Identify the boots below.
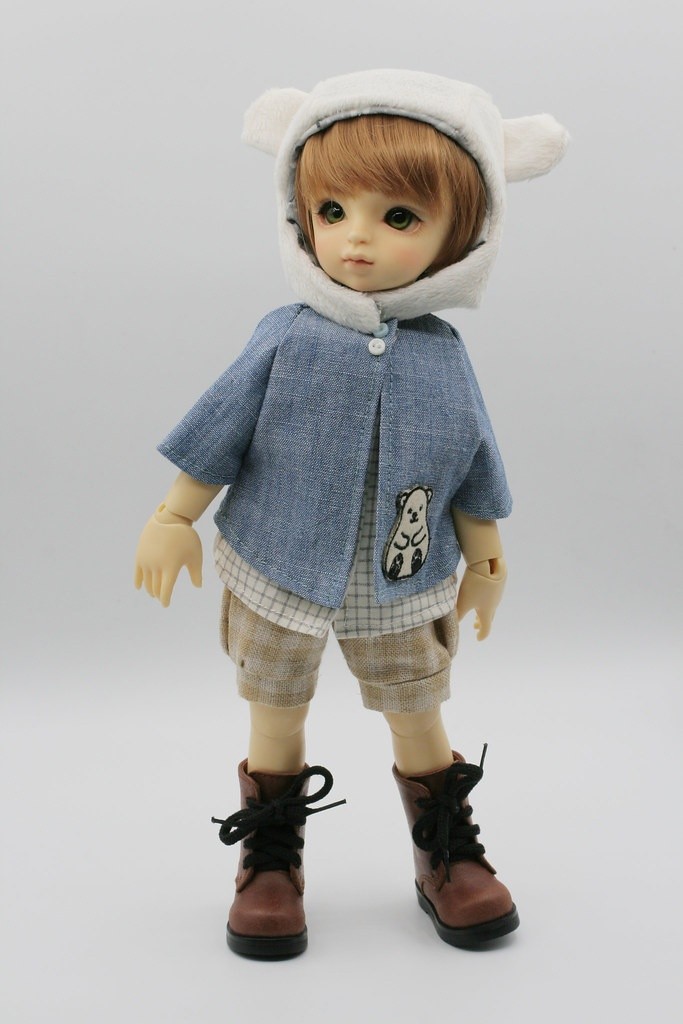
[391,743,520,948]
[210,759,347,960]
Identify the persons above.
[134,67,570,963]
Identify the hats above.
[241,67,568,334]
